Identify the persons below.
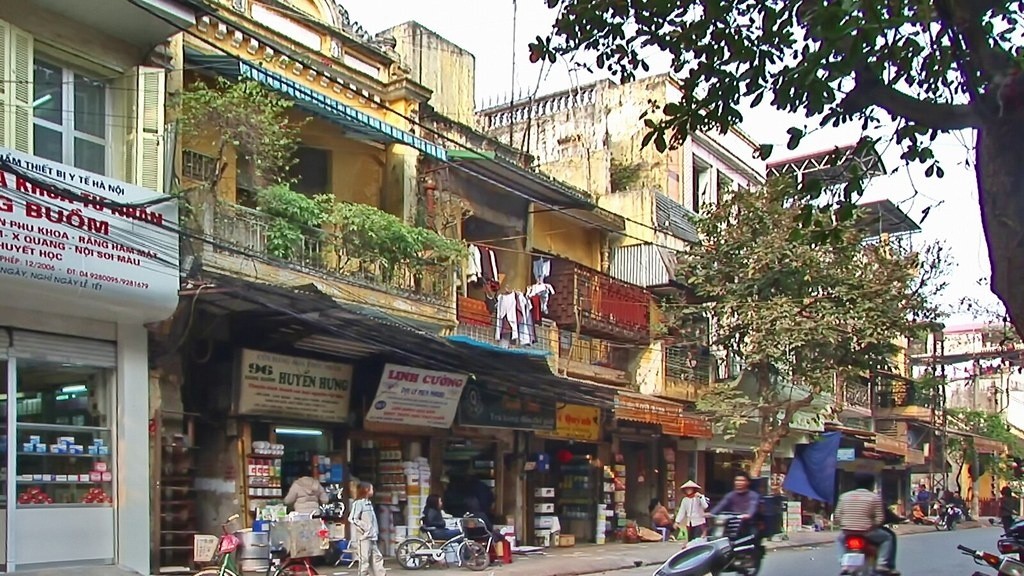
[997,486,1018,543]
[703,472,759,565]
[348,481,387,576]
[283,466,329,516]
[834,472,895,574]
[649,498,680,532]
[917,484,930,516]
[422,495,470,560]
[673,479,710,543]
[465,496,496,566]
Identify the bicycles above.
[935,504,959,530]
[190,514,321,576]
[396,512,492,570]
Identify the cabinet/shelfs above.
[15,421,112,509]
[556,453,595,519]
[247,453,283,512]
[154,409,200,576]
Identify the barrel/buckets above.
[596,503,607,544]
[235,532,269,571]
[378,456,432,559]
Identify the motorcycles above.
[839,535,880,576]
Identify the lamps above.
[274,427,322,436]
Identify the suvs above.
[702,514,766,576]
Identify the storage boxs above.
[535,487,555,497]
[534,503,554,513]
[534,516,553,528]
[559,534,575,547]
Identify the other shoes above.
[876,564,890,571]
[744,559,754,566]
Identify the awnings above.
[187,53,448,161]
[917,420,1007,454]
[449,151,599,209]
[780,433,841,503]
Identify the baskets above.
[193,533,221,564]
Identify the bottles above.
[558,473,587,519]
[360,439,407,504]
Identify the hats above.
[680,480,702,489]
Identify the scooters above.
[957,544,1024,576]
[997,519,1024,563]
[652,534,754,576]
